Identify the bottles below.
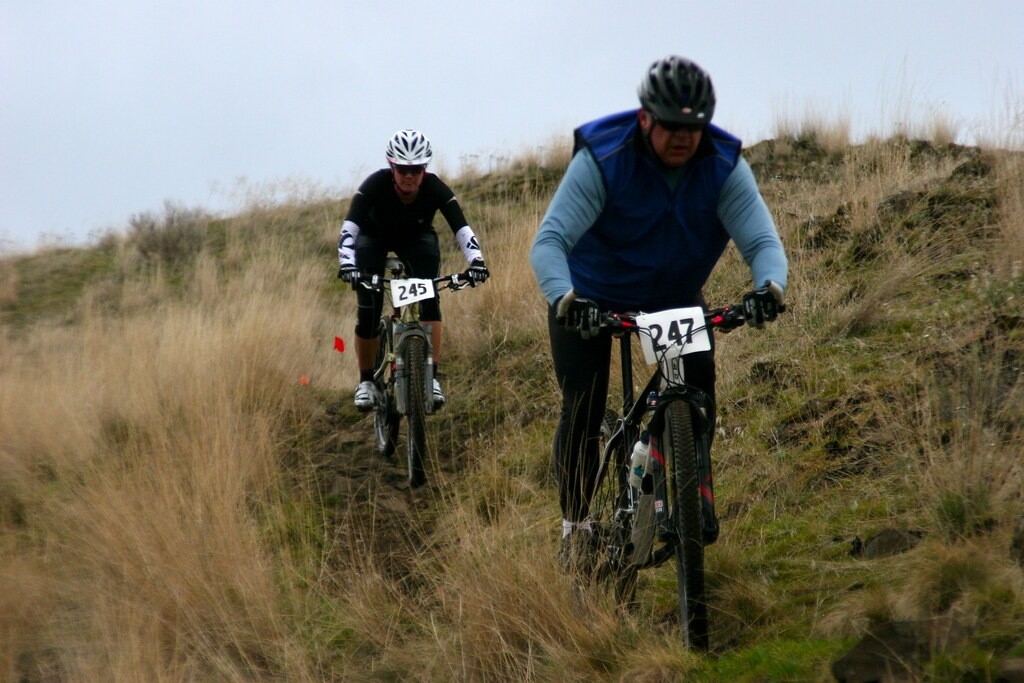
[629,429,650,488]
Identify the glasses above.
[644,106,710,134]
[390,163,425,176]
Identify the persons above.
[529,54,790,578]
[338,130,490,411]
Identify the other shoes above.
[559,526,594,572]
[658,516,682,544]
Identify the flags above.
[334,336,344,352]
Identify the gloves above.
[465,264,489,288]
[339,264,362,291]
[563,298,600,339]
[743,286,780,331]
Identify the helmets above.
[386,128,432,165]
[639,55,715,124]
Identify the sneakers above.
[353,380,377,408]
[423,378,446,405]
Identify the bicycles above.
[348,260,479,490]
[567,300,787,655]
